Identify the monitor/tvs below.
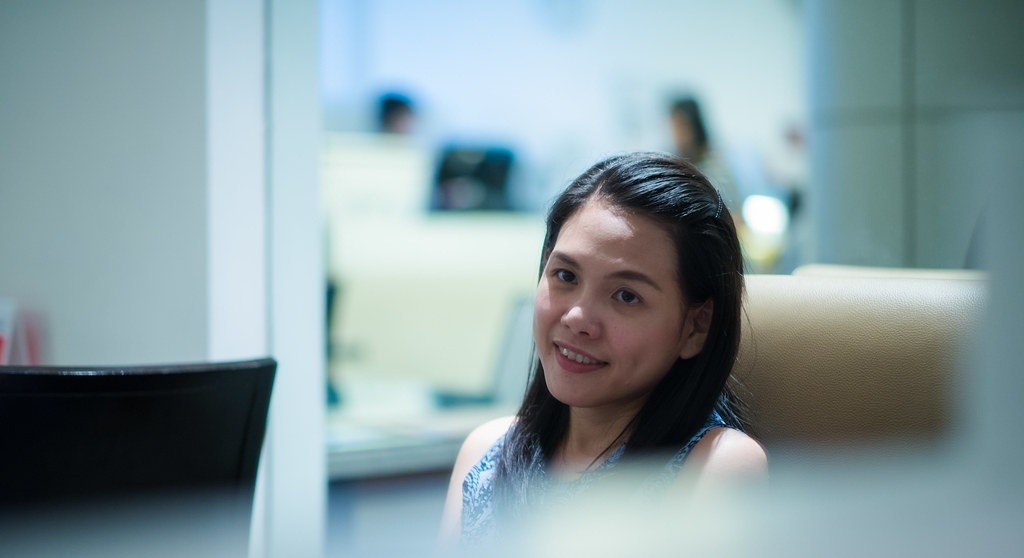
[0,357,278,558]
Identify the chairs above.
[697,270,998,557]
[1,358,277,558]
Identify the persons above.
[374,95,416,134]
[440,150,769,558]
[664,89,742,228]
